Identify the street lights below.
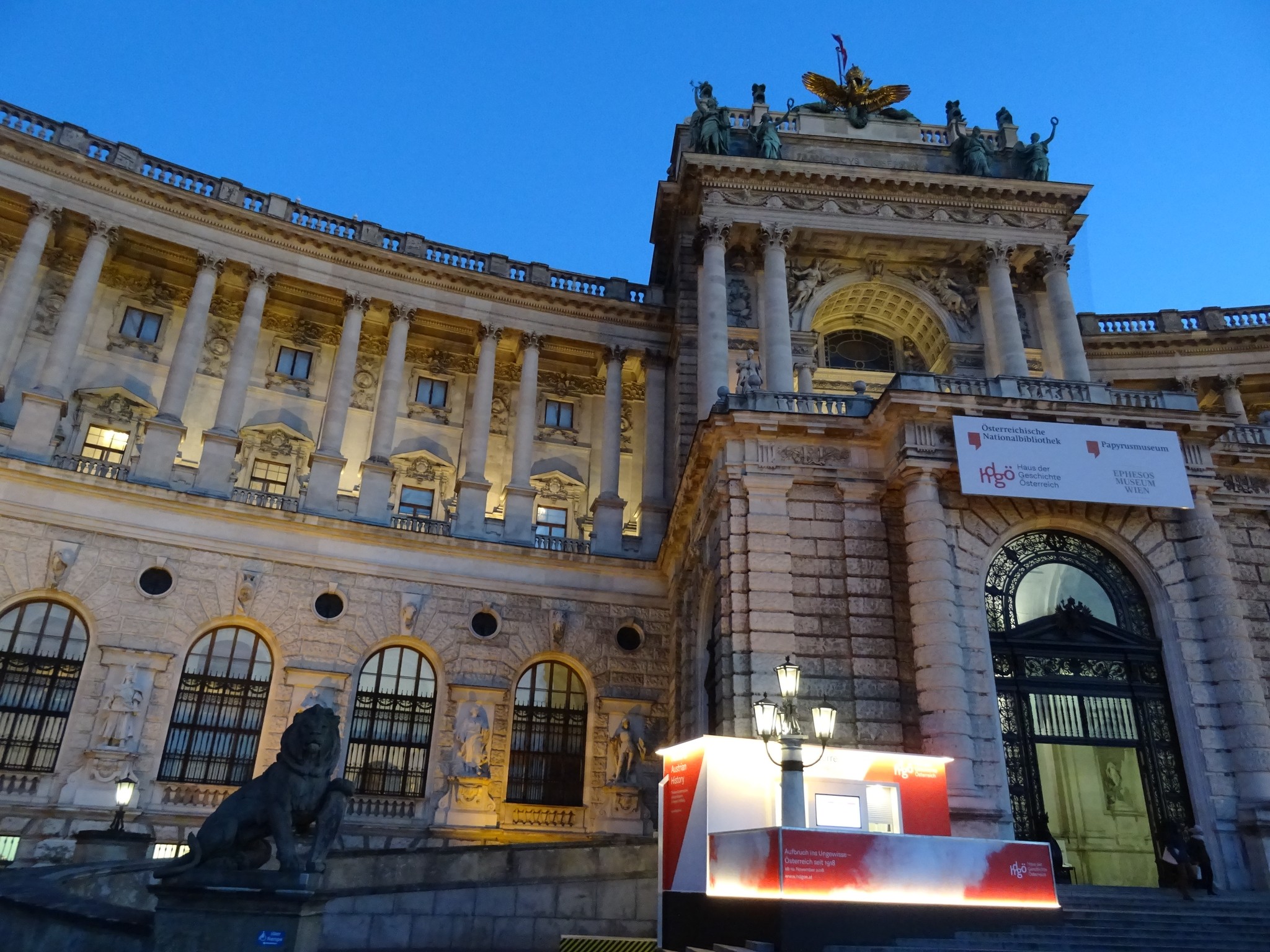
[752,656,838,830]
[105,772,137,830]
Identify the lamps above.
[106,771,137,833]
[752,654,837,831]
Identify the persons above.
[1165,824,1195,900]
[97,670,143,748]
[757,104,791,160]
[735,349,762,393]
[913,267,969,313]
[736,358,750,385]
[1026,124,1056,181]
[694,87,727,154]
[786,260,841,314]
[1189,825,1217,896]
[611,717,637,785]
[456,708,486,775]
[955,117,994,177]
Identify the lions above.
[152,703,357,879]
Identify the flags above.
[831,33,847,72]
